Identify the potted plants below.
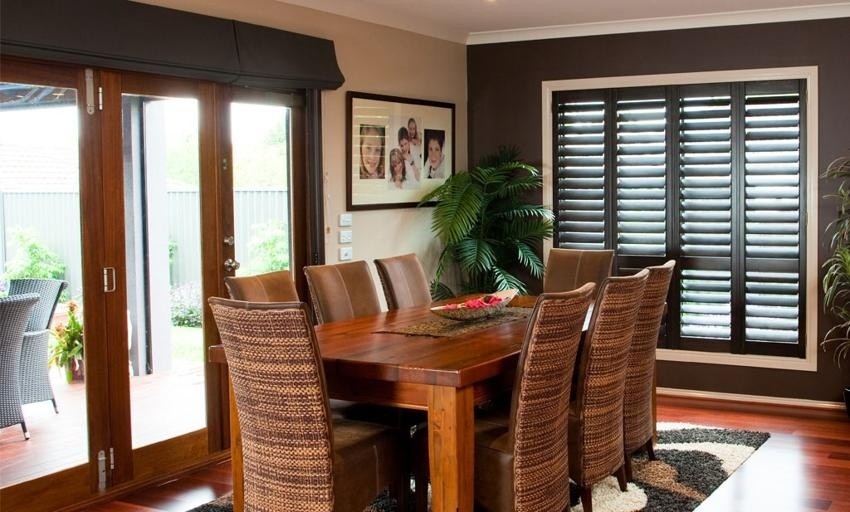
[818,155,850,418]
[49,300,87,385]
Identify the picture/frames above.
[344,90,460,211]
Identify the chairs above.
[0,291,41,440]
[221,252,435,337]
[9,279,68,413]
[541,246,623,297]
[205,296,401,512]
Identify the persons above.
[408,118,422,144]
[360,124,385,179]
[398,127,421,181]
[390,148,405,187]
[424,129,445,178]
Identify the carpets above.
[185,418,771,512]
